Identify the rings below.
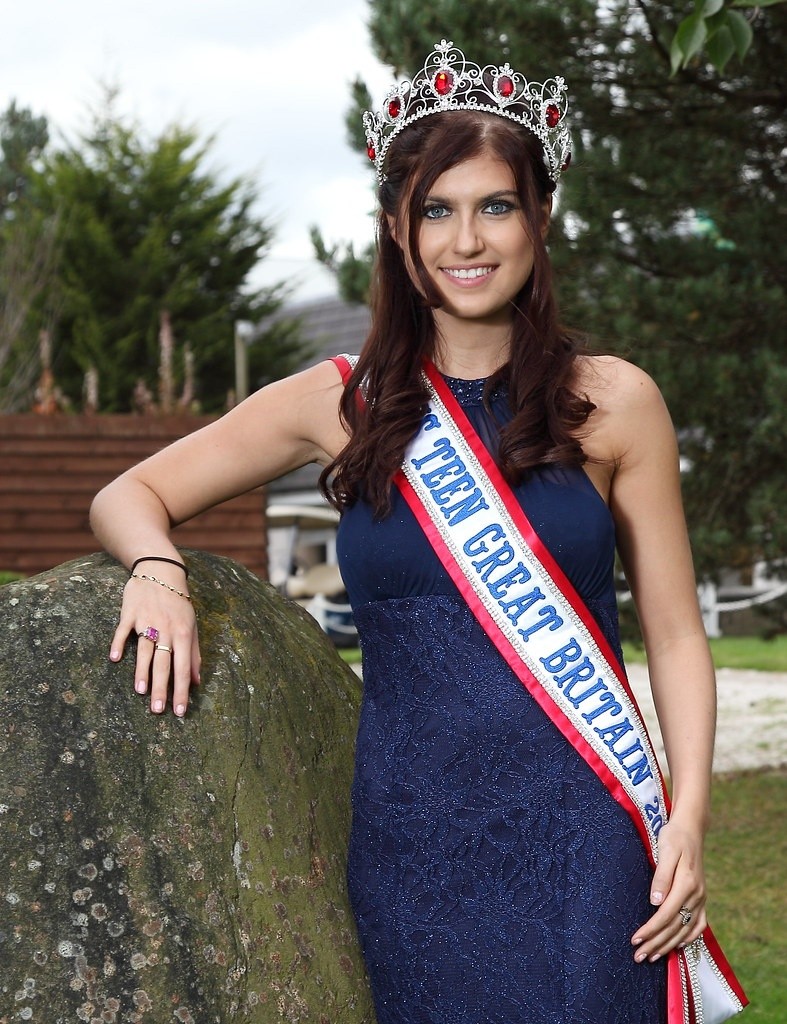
[139,626,158,642]
[679,907,692,925]
[154,645,172,654]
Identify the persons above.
[88,38,717,1024]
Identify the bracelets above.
[130,573,192,601]
[130,556,189,581]
